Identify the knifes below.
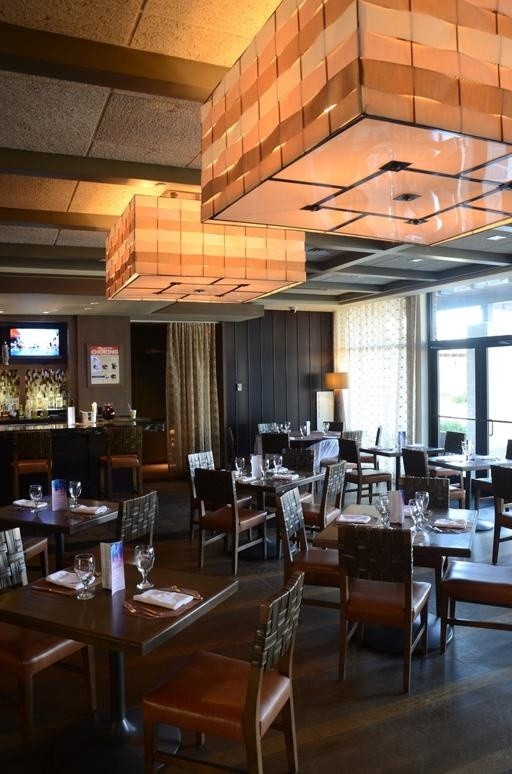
[31,582,74,595]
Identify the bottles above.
[103,405,115,419]
[1,368,68,418]
[91,402,98,416]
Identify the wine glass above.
[30,485,44,514]
[272,455,282,470]
[274,420,330,434]
[372,489,430,532]
[69,481,81,509]
[234,457,245,478]
[75,554,96,602]
[460,440,475,462]
[260,458,269,479]
[134,545,155,590]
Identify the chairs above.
[275,485,341,608]
[335,522,433,695]
[440,559,512,656]
[2,418,512,576]
[2,526,97,741]
[141,568,305,772]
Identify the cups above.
[91,412,96,422]
[98,407,102,414]
[80,412,88,421]
[129,410,137,419]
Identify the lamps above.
[324,370,351,394]
[97,185,310,310]
[188,0,512,255]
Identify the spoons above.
[122,600,162,618]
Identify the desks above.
[311,503,480,659]
[2,554,240,773]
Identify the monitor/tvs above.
[6,323,70,367]
